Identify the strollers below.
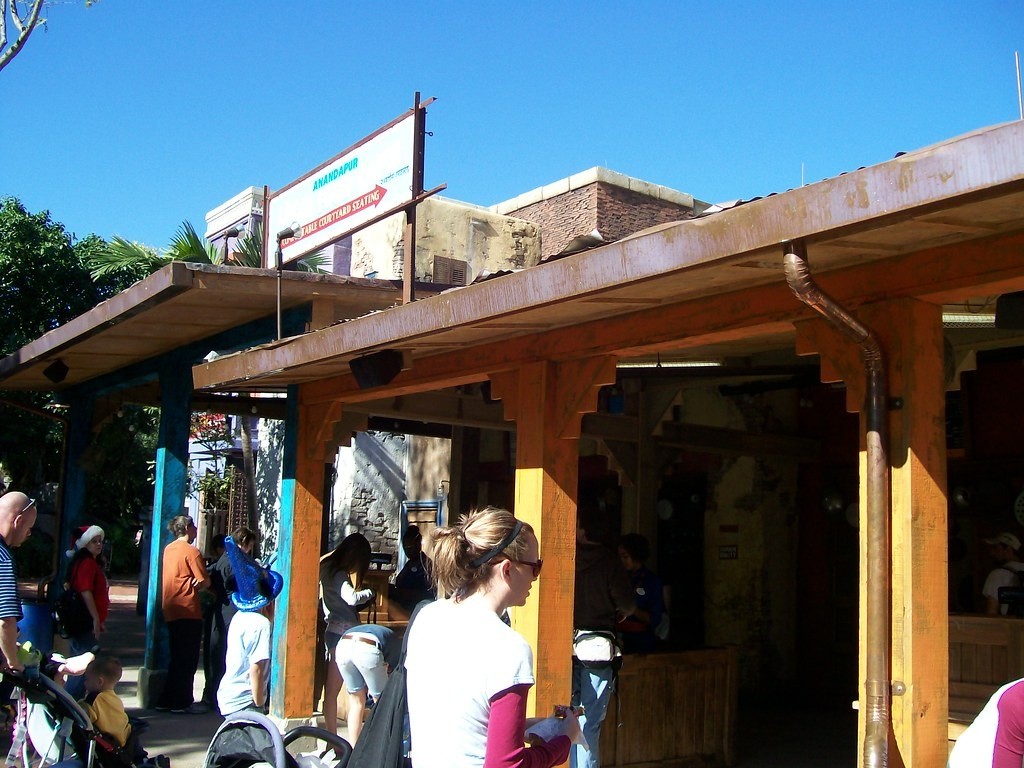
[2,641,152,768]
[204,712,353,768]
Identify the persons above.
[155,516,211,714]
[217,536,284,721]
[319,533,376,751]
[405,505,589,768]
[982,532,1024,617]
[0,491,132,768]
[570,512,672,768]
[945,678,1024,768]
[335,623,401,749]
[388,525,438,618]
[201,527,257,712]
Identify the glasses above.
[490,558,543,577]
[13,498,38,523]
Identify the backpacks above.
[52,556,97,640]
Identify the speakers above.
[347,347,406,391]
[42,358,71,383]
[994,289,1024,329]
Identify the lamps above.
[43,362,68,383]
[349,347,415,388]
[275,222,303,339]
[220,223,247,263]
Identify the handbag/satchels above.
[346,598,434,768]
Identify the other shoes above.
[154,704,188,713]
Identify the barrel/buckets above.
[15,597,54,657]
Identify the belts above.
[342,634,381,650]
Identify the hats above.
[65,524,105,559]
[224,536,284,612]
